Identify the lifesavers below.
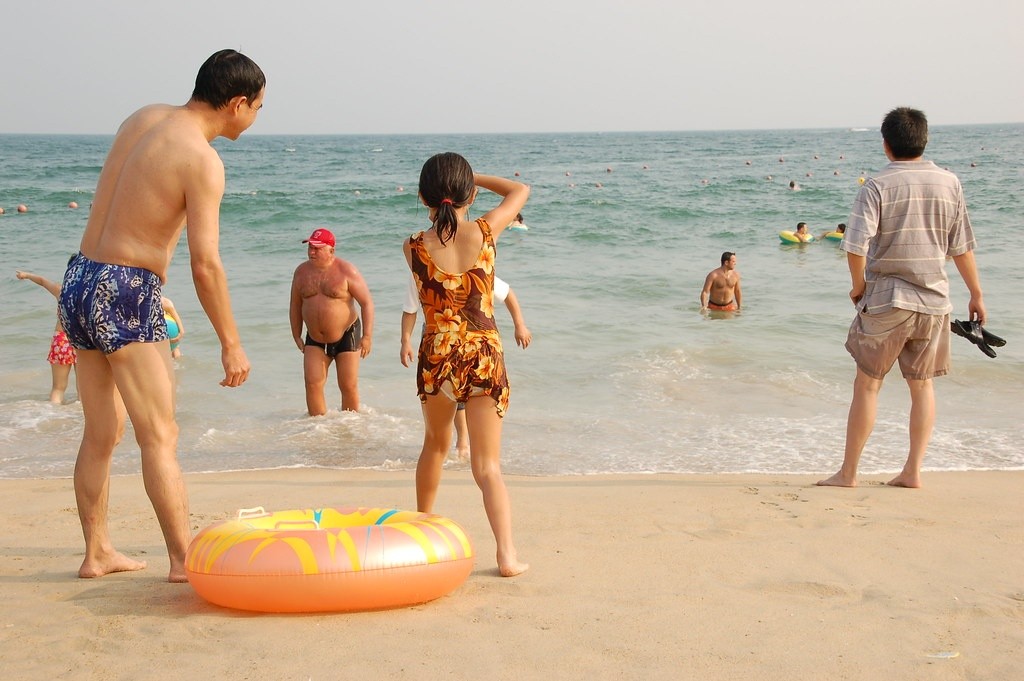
[506,222,528,231]
[777,229,813,247]
[182,507,472,613]
[163,315,181,350]
[824,232,844,242]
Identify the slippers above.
[951,319,1006,358]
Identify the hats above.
[301,229,336,249]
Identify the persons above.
[290,229,373,417]
[700,251,742,309]
[160,298,184,359]
[59,49,266,583]
[403,152,530,574]
[16,270,80,403]
[817,107,986,488]
[400,268,532,449]
[0,202,79,214]
[354,155,978,243]
